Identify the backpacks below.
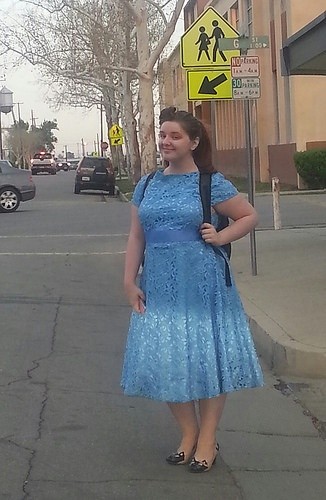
[144,172,231,260]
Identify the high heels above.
[166,443,197,465]
[189,441,220,473]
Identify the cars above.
[0,163,35,213]
[57,159,78,172]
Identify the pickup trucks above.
[31,153,58,174]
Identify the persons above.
[123,107,265,473]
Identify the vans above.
[74,156,116,194]
